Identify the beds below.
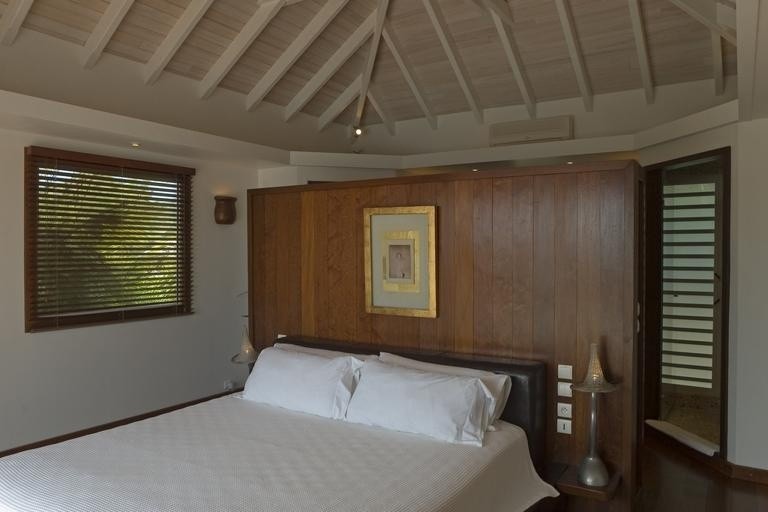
[0,334,546,512]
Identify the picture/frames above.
[362,205,437,319]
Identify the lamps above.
[214,195,238,224]
[230,326,260,374]
[570,341,620,487]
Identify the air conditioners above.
[489,115,574,146]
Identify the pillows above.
[379,351,512,432]
[243,347,364,421]
[345,358,496,448]
[273,343,379,394]
